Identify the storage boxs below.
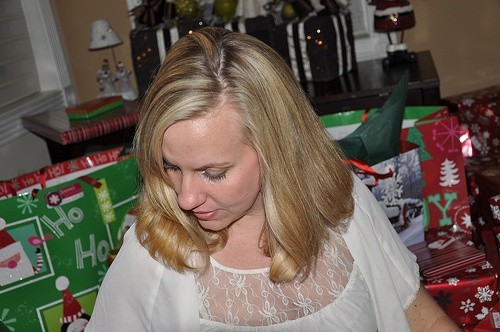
[65,95,124,120]
[406,85,500,332]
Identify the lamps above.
[88,19,138,101]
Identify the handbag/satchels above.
[0,146,142,332]
[317,105,474,248]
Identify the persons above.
[83,27,460,332]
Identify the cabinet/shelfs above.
[299,49,442,117]
[21,100,142,165]
